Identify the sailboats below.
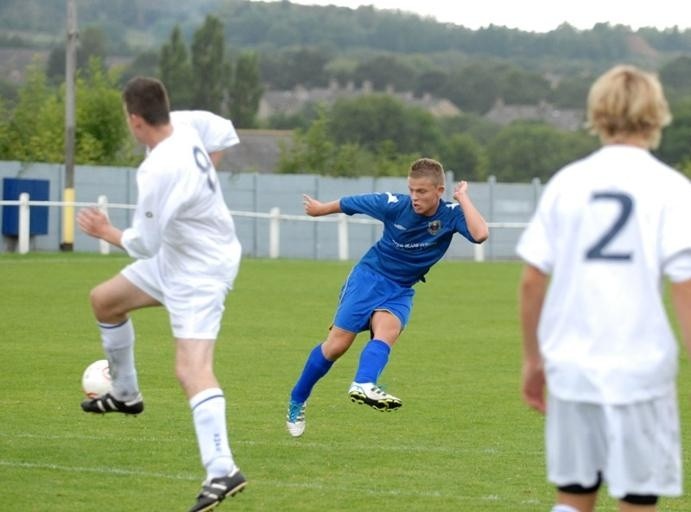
[81,359,113,401]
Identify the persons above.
[77,76,248,512]
[515,65,690,511]
[287,158,489,437]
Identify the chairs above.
[189,469,246,512]
[287,400,306,437]
[80,392,144,414]
[348,382,403,412]
